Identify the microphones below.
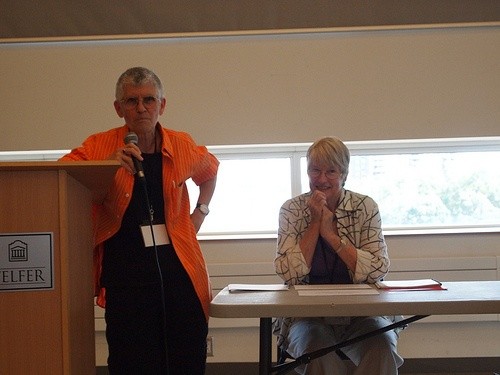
[123,132,146,181]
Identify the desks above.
[210,280,500,375]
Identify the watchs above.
[336,238,347,253]
[196,203,209,215]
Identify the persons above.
[273,137,403,375]
[56,67,219,374]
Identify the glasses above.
[122,95,162,110]
[307,167,343,180]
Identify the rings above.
[121,147,126,154]
[121,154,126,161]
